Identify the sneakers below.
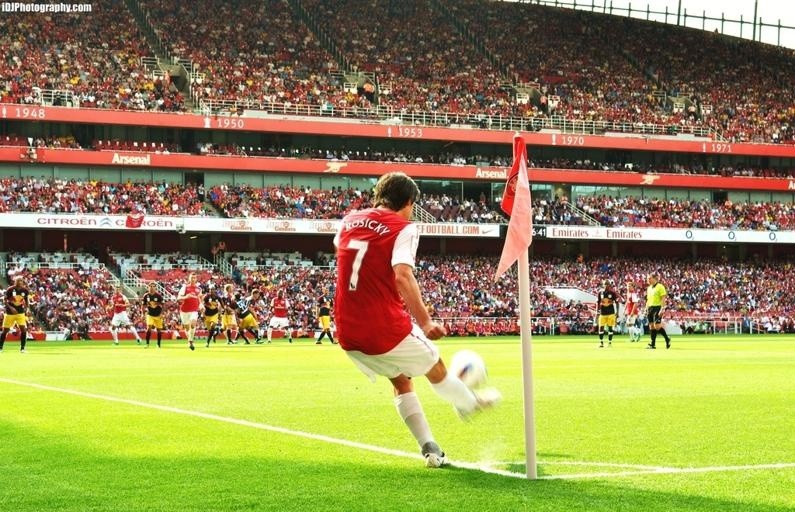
[0,336,341,354]
[599,336,642,350]
[425,448,450,469]
[665,335,672,350]
[644,343,657,350]
[454,384,501,425]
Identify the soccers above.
[451,351,485,386]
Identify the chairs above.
[0,0,795,337]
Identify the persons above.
[1,1,795,352]
[333,171,499,468]
